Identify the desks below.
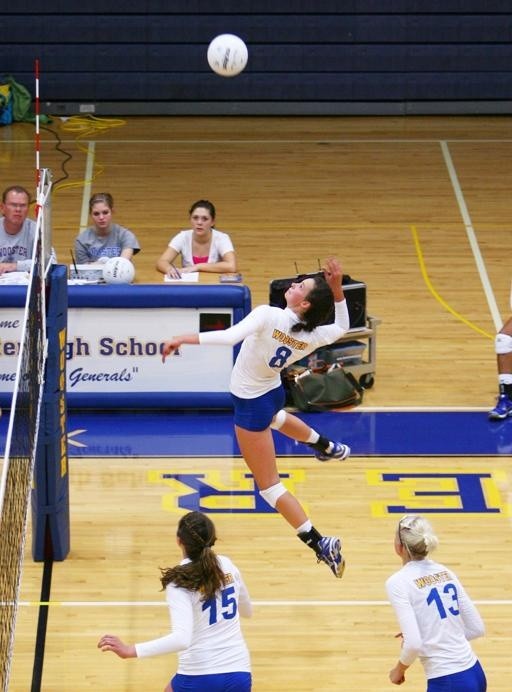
[1,270,251,409]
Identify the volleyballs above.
[102,257,134,285]
[208,33,248,77]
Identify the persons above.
[156,199,237,280]
[96,513,254,692]
[384,515,487,692]
[74,192,141,266]
[161,257,351,578]
[488,315,512,422]
[0,186,57,277]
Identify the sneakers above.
[316,442,351,462]
[317,536,346,579]
[489,394,512,420]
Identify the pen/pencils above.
[71,249,77,274]
[172,264,181,279]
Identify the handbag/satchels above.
[289,359,364,412]
